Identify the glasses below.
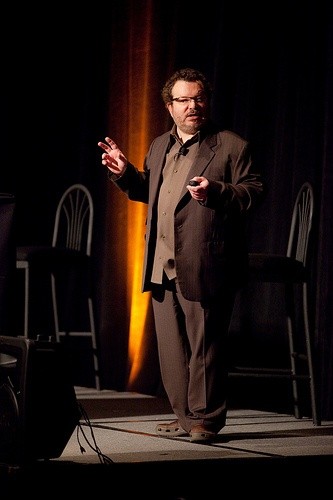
[172,96,208,103]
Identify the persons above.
[99,69,262,441]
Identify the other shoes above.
[190,426,216,442]
[155,420,188,436]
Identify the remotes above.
[189,181,198,186]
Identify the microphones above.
[180,147,186,154]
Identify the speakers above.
[0,336,82,460]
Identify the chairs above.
[223,182,321,427]
[15,184,104,391]
